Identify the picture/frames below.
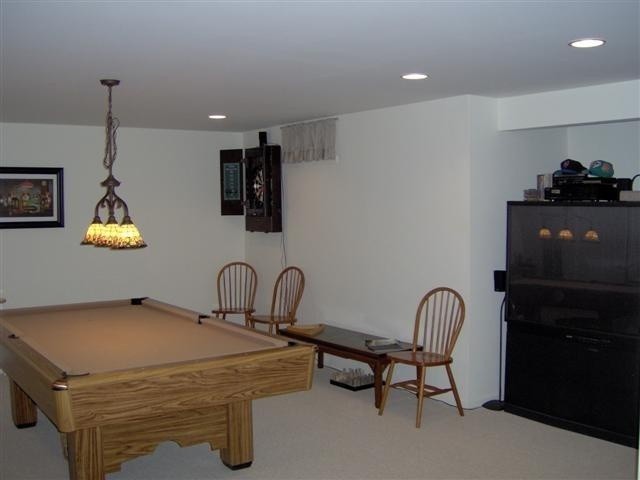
[0,167,65,228]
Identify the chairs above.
[249,263,306,336]
[377,288,465,426]
[211,264,259,324]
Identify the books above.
[365,337,401,346]
[369,343,404,352]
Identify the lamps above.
[80,78,148,250]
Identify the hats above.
[560,159,614,178]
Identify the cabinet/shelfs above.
[504,321,640,450]
[239,143,283,233]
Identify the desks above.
[0,296,318,480]
[281,322,424,407]
